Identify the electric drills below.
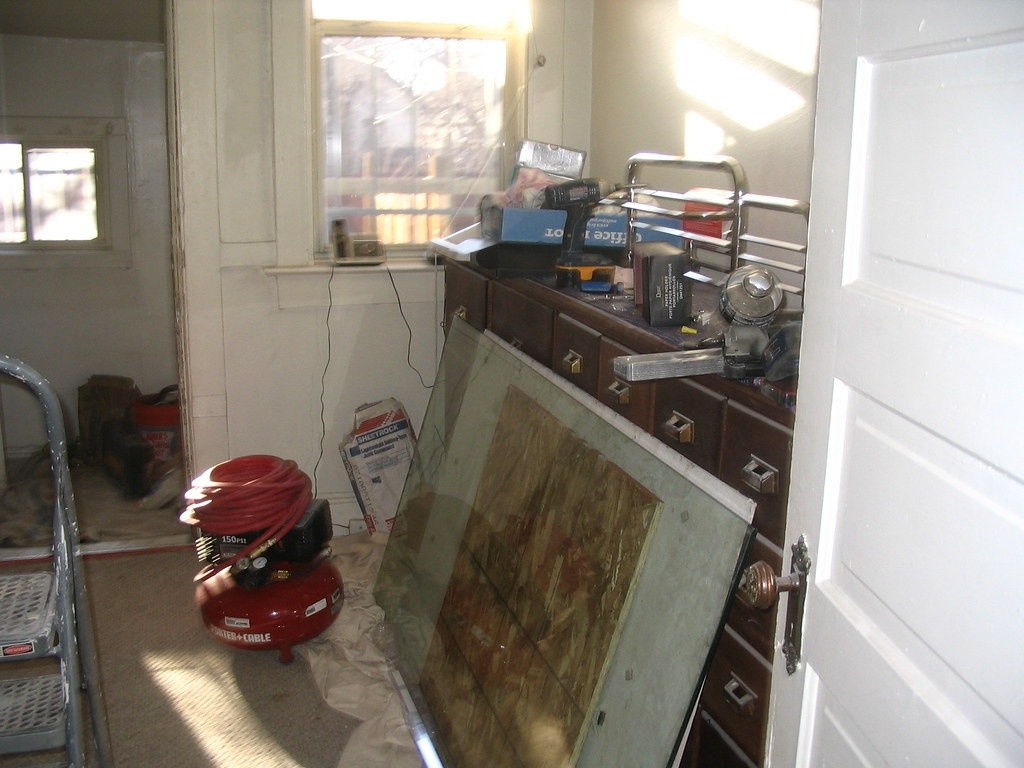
[545,177,648,284]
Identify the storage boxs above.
[556,266,634,288]
[499,209,684,250]
[634,243,693,327]
[339,397,417,535]
[512,139,586,192]
[683,189,741,242]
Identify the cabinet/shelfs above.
[441,256,801,768]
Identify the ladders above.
[0,355,116,768]
